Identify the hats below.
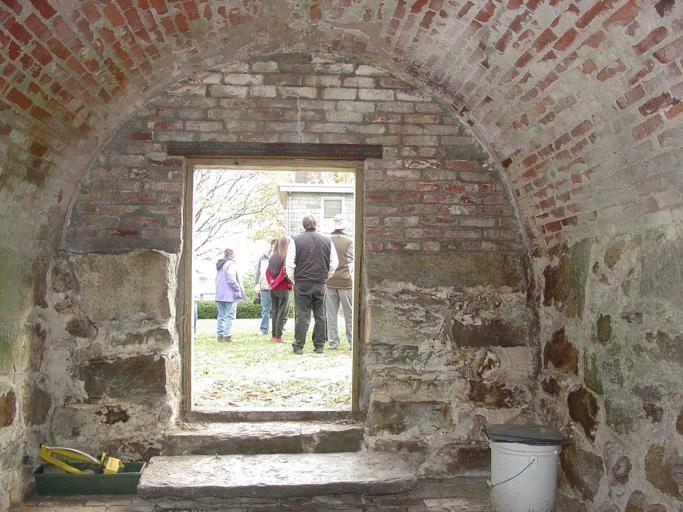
[332,214,346,230]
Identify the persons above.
[265,235,292,343]
[284,214,340,355]
[254,239,277,336]
[213,248,246,342]
[325,213,352,350]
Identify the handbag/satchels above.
[253,291,261,305]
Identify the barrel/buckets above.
[488,441,561,511]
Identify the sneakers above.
[273,338,287,343]
[313,346,322,352]
[255,330,268,336]
[293,347,302,354]
